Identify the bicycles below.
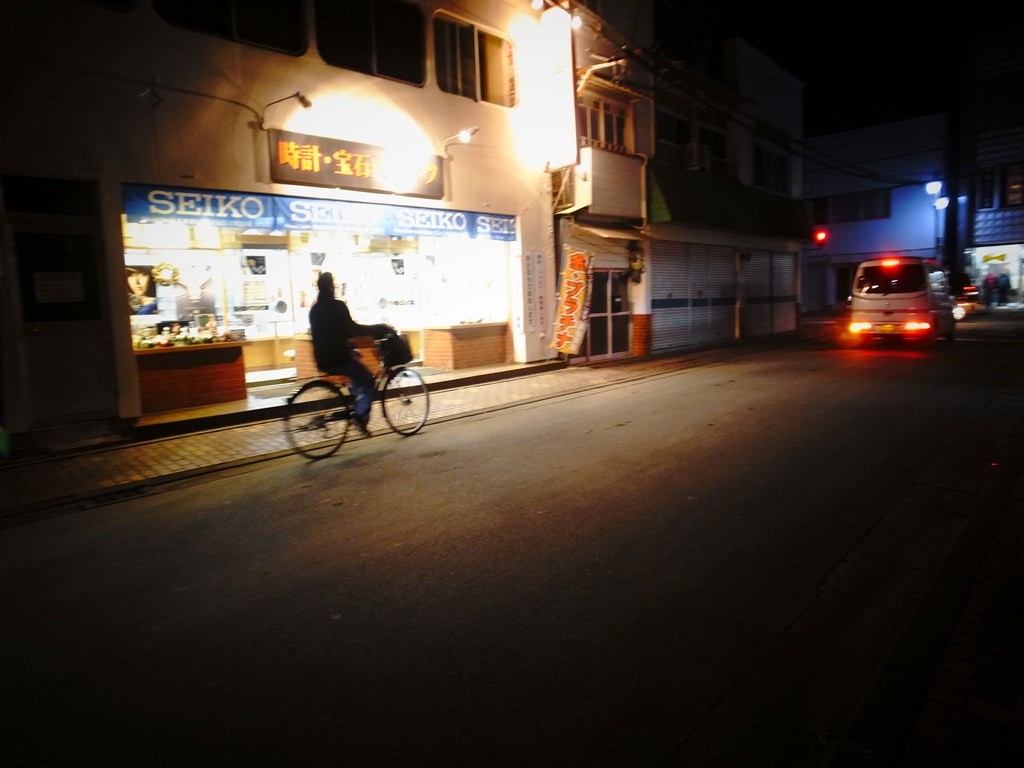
[283,328,430,460]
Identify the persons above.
[125,265,157,315]
[983,273,1010,306]
[309,272,396,439]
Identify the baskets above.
[380,334,413,367]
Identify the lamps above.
[264,91,312,108]
[441,125,479,161]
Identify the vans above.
[849,256,955,345]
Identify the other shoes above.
[347,421,372,440]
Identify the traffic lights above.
[816,227,826,246]
